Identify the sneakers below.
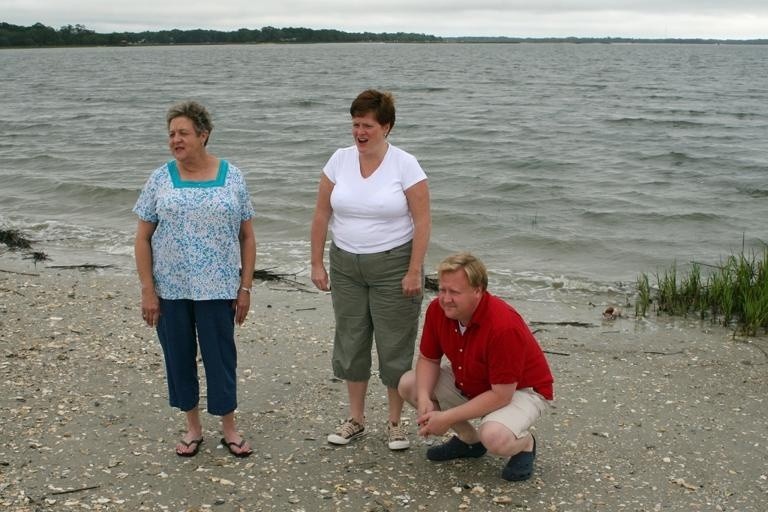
[386,419,410,451]
[500,433,537,482]
[326,416,367,445]
[426,435,488,461]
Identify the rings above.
[143,314,146,318]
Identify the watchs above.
[240,286,253,294]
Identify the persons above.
[131,101,256,458]
[398,254,554,480]
[310,88,431,451]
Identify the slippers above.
[176,437,204,457]
[220,437,253,458]
